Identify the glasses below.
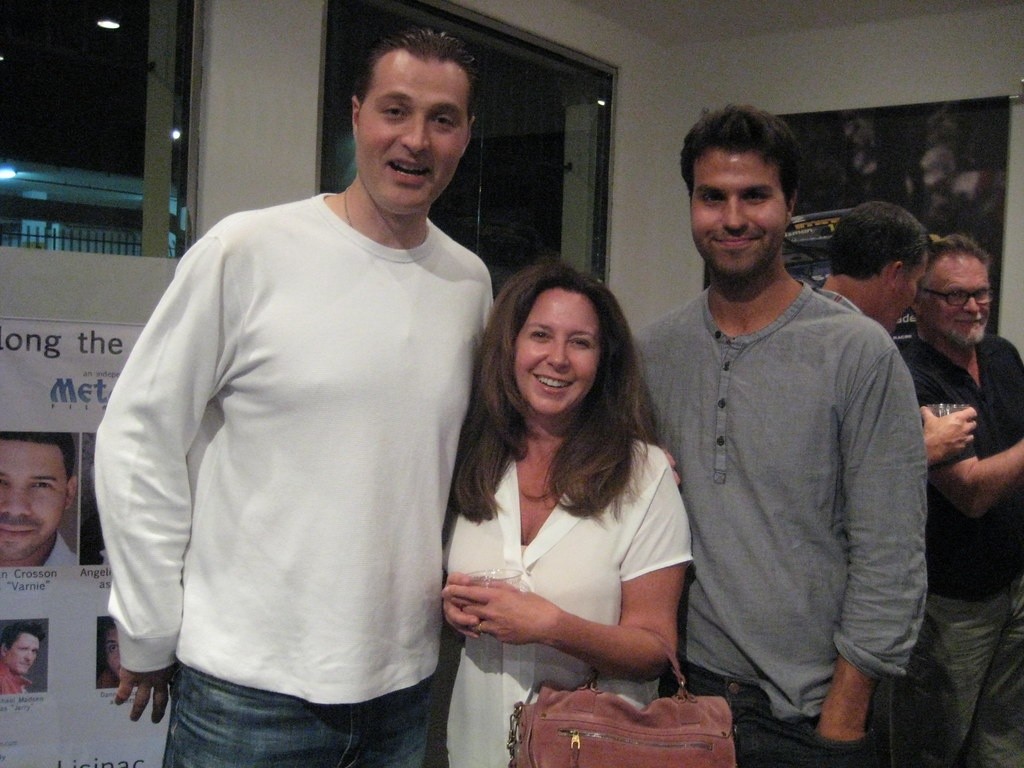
[923,288,994,307]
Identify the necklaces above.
[344,187,353,228]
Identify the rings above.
[476,622,487,634]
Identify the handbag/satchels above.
[505,623,737,768]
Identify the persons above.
[97,624,122,688]
[890,233,1024,768]
[633,102,927,768]
[0,620,46,694]
[0,431,77,566]
[443,264,694,767]
[94,30,493,768]
[803,201,977,467]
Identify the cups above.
[927,404,969,456]
[461,569,523,639]
